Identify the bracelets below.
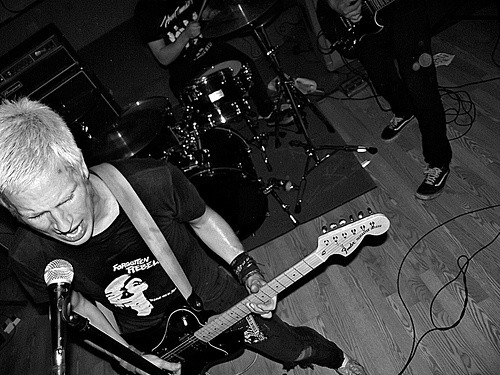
[229,251,261,283]
[121,344,145,374]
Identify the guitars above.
[316,0,394,60]
[150,207,391,375]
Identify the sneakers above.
[416,164,450,200]
[381,113,417,141]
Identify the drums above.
[198,61,254,90]
[179,67,251,128]
[122,97,173,127]
[162,127,269,242]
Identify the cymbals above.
[93,108,162,162]
[201,0,275,39]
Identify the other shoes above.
[266,110,296,127]
[334,352,368,375]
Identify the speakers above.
[32,64,122,168]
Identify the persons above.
[139,0,295,126]
[0,96,368,375]
[314,0,453,199]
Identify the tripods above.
[232,0,378,214]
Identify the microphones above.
[44,258,74,375]
